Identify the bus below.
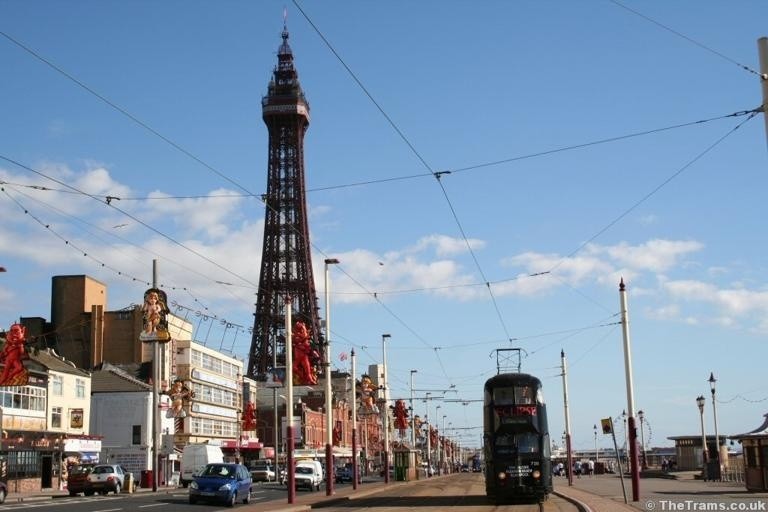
[484,373,553,503]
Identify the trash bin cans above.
[141,469,153,488]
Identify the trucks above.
[473,455,481,472]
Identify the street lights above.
[707,372,721,454]
[381,332,391,483]
[324,257,340,494]
[695,395,707,457]
[406,369,458,481]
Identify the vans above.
[294,459,323,491]
[179,446,227,487]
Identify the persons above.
[332,374,444,452]
[587,457,595,477]
[575,458,582,479]
[143,292,161,334]
[558,461,563,476]
[0,324,26,384]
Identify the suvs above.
[189,464,254,506]
[336,463,363,485]
[461,464,469,472]
[68,463,96,497]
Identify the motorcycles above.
[278,468,287,486]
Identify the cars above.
[248,459,274,482]
[86,464,136,494]
[0,482,6,506]
